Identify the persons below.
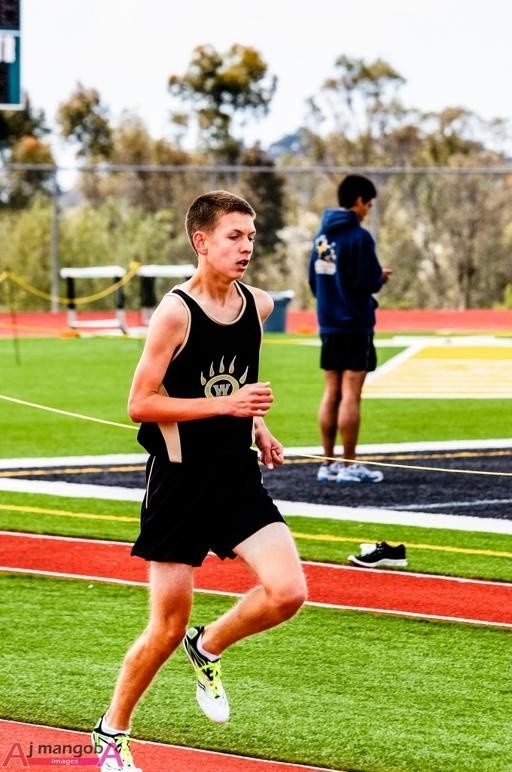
[91,189,309,771]
[307,175,392,485]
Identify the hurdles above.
[136,264,196,326]
[60,265,128,335]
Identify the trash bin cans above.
[263,297,291,332]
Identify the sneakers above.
[347,541,408,568]
[91,713,137,772]
[316,461,384,484]
[183,626,230,723]
